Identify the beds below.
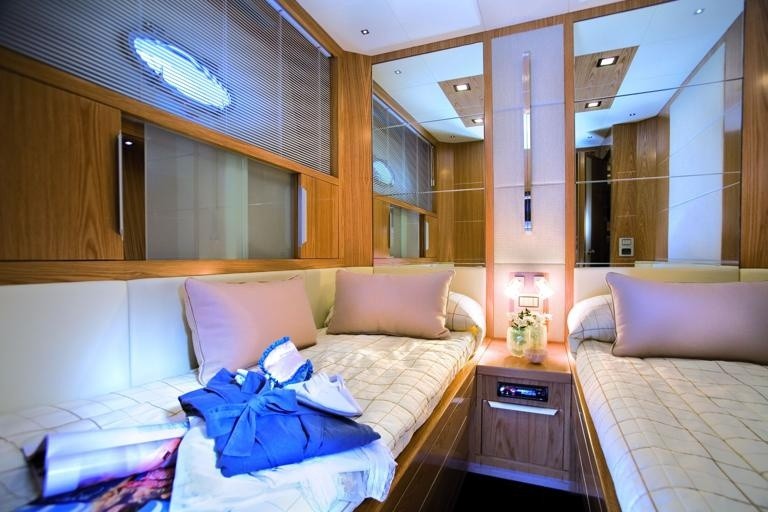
[566,337,768,511]
[2,330,483,512]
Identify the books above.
[18,420,190,496]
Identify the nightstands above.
[470,337,573,481]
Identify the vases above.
[507,327,548,363]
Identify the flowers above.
[508,308,553,329]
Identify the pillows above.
[322,268,485,344]
[566,271,767,364]
[181,274,318,381]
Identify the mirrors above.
[370,41,486,267]
[571,1,745,267]
